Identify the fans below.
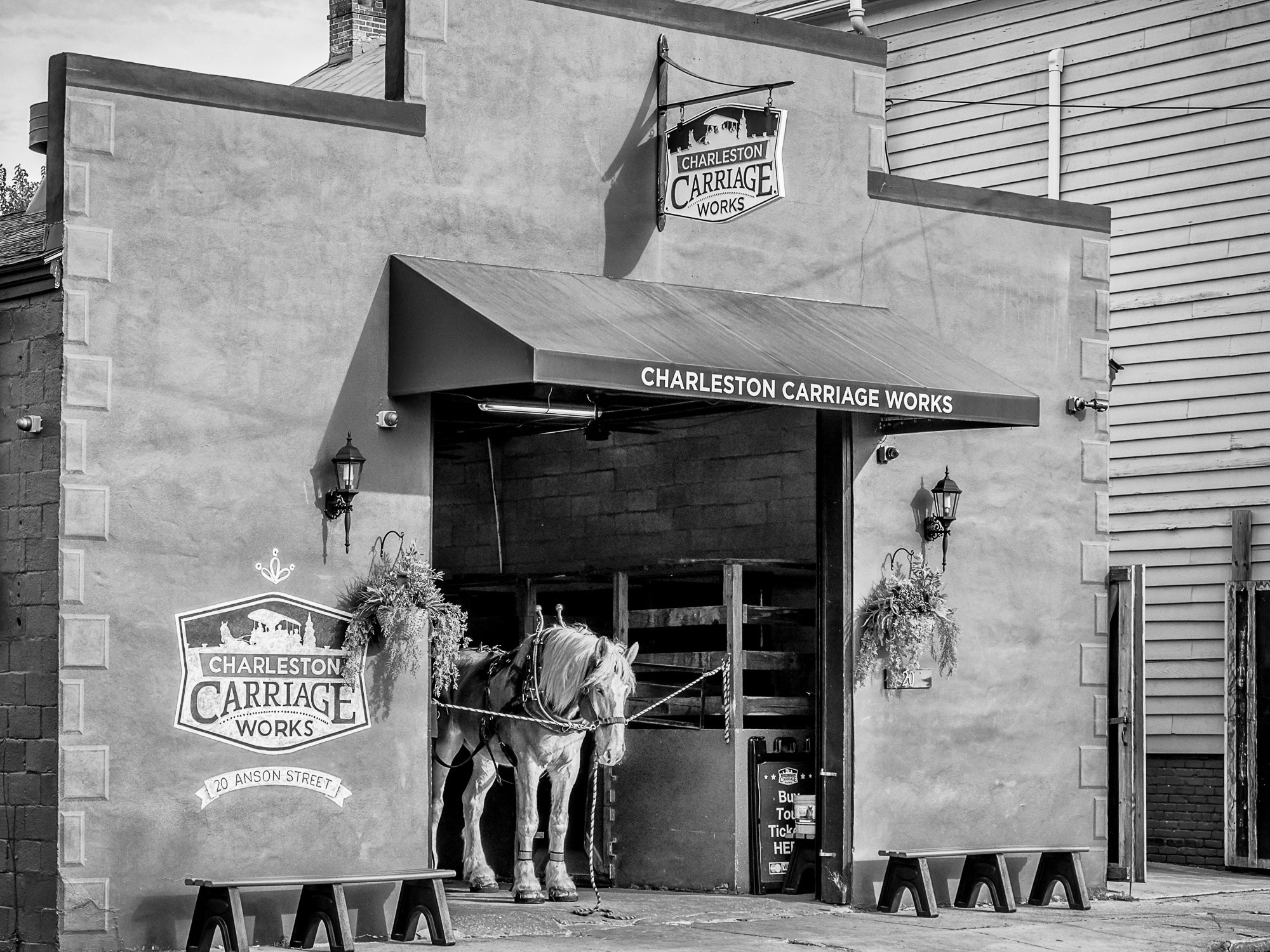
[525,390,661,441]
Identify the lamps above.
[924,465,962,571]
[478,386,603,418]
[325,430,366,554]
[1069,396,1109,413]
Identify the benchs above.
[876,845,1091,917]
[185,869,456,952]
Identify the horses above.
[428,622,641,902]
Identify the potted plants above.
[342,539,473,698]
[853,551,962,697]
